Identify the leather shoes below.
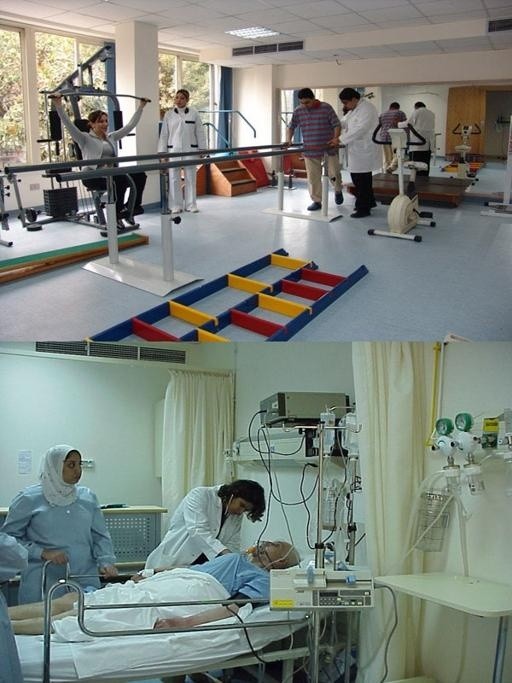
[351,212,370,217]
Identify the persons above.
[284,87,344,211]
[1,443,120,604]
[0,528,29,683]
[398,101,436,174]
[54,93,150,229]
[155,88,209,214]
[1,537,302,636]
[378,100,407,174]
[327,85,379,218]
[145,479,265,571]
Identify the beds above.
[0,555,370,683]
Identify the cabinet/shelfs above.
[374,568,511,683]
[0,501,169,582]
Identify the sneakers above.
[387,168,392,174]
[125,218,135,225]
[117,220,125,231]
[335,191,343,204]
[308,202,321,210]
[172,209,182,213]
[186,208,199,213]
